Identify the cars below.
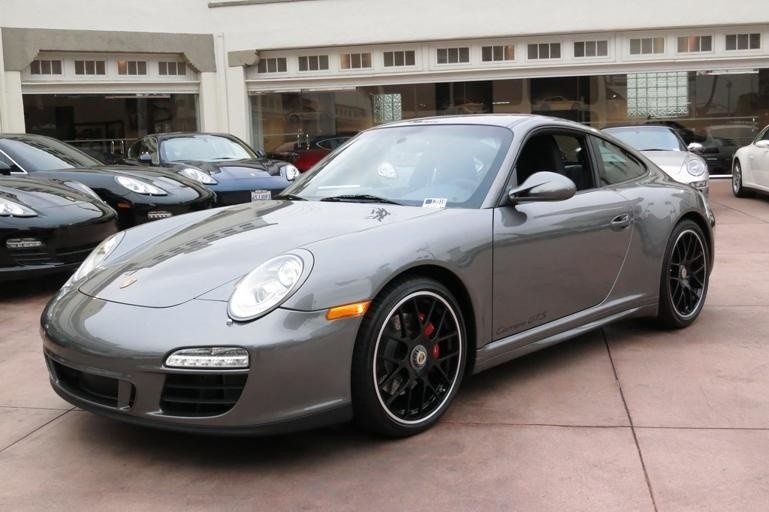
[533,96,582,111]
